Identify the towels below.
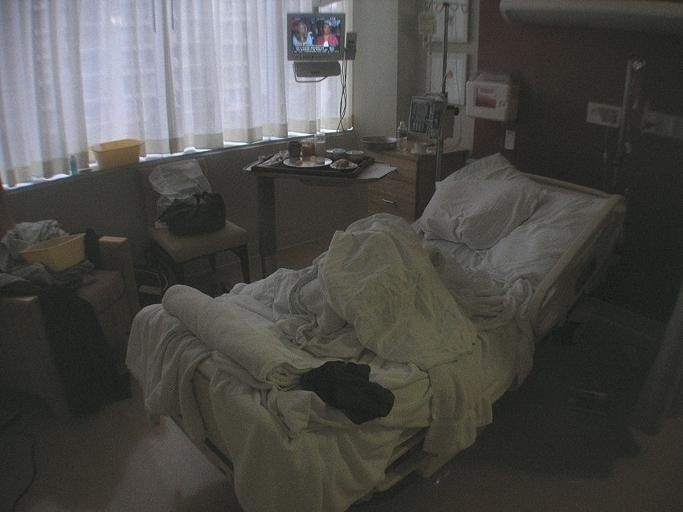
[156,284,314,388]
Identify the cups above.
[289,132,326,157]
[415,143,427,152]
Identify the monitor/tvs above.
[287,13,345,59]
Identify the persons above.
[291,18,316,52]
[316,22,340,47]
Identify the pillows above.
[418,174,544,255]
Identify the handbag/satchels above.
[161,192,226,236]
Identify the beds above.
[122,159,628,512]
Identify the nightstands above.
[364,141,466,220]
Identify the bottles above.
[396,121,408,152]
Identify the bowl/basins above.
[359,136,397,151]
[326,148,365,163]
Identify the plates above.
[330,161,358,170]
[410,149,432,154]
[282,156,332,167]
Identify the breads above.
[335,158,349,168]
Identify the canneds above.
[288,141,300,163]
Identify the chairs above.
[0,222,139,421]
[139,157,250,294]
